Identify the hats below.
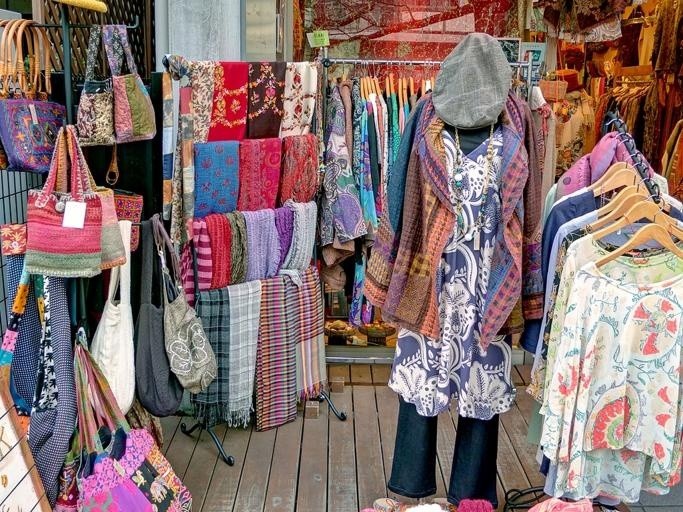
[554,69,585,92]
[431,32,512,130]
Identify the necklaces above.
[452,125,494,239]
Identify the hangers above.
[326,58,441,101]
[587,117,683,269]
[609,66,661,101]
[508,62,540,98]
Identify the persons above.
[360,29,541,509]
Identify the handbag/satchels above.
[87,220,137,420]
[151,213,218,395]
[76,24,123,148]
[53,327,193,512]
[538,80,567,102]
[23,125,143,278]
[102,24,157,145]
[1,18,67,173]
[134,220,184,417]
